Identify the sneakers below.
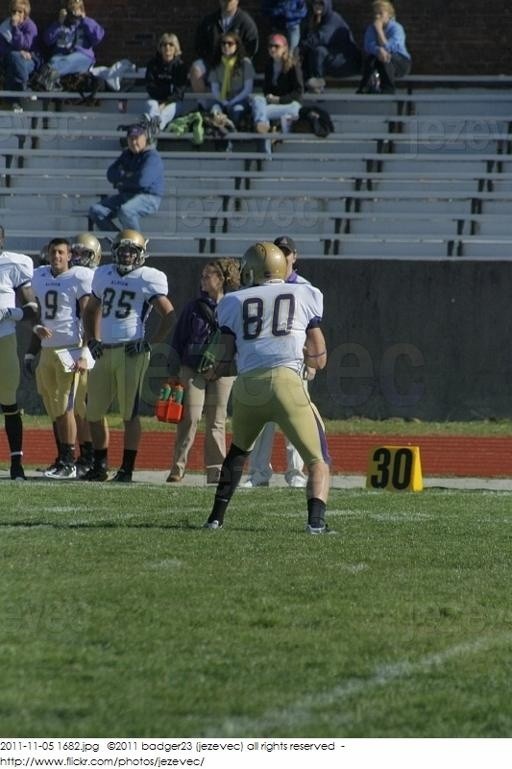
[166,471,184,481]
[11,465,26,479]
[44,458,131,482]
[306,523,326,535]
[204,520,222,529]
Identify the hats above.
[275,237,296,251]
[128,126,145,137]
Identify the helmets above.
[70,234,101,268]
[241,242,286,286]
[112,231,145,271]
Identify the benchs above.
[0,70,512,261]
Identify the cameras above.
[68,9,76,17]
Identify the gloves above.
[125,340,151,357]
[86,338,104,359]
[23,356,35,378]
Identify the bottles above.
[173,385,184,403]
[370,68,380,90]
[159,382,171,402]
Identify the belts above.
[101,343,125,348]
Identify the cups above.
[280,115,292,135]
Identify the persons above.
[188,0,260,94]
[364,0,412,95]
[253,33,304,133]
[209,31,255,131]
[301,0,362,81]
[1,226,332,537]
[272,0,307,58]
[144,33,185,131]
[0,0,45,112]
[41,0,106,78]
[89,126,166,231]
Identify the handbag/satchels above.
[300,106,334,136]
[32,63,105,92]
